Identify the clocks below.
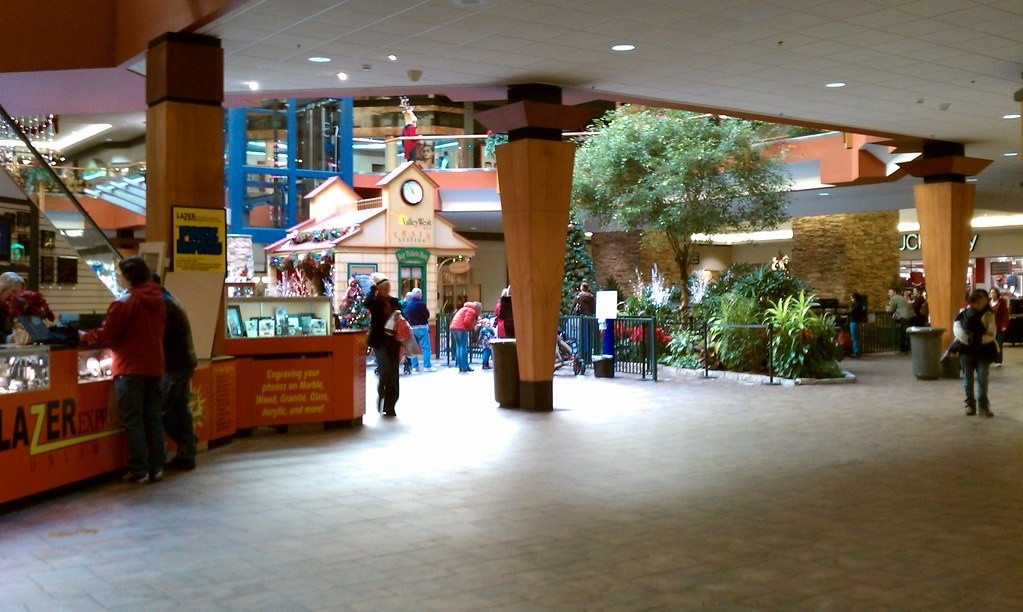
[400,179,424,205]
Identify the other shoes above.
[123,472,149,483]
[424,367,437,372]
[149,469,162,481]
[386,410,396,416]
[979,406,994,417]
[459,367,474,372]
[412,368,418,372]
[966,406,976,416]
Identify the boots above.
[482,362,492,369]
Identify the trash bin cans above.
[906,327,945,380]
[592,354,613,378]
[488,339,520,408]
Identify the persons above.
[402,123,423,162]
[398,288,437,372]
[363,272,404,416]
[79,257,195,478]
[571,283,594,315]
[496,285,515,338]
[941,287,1007,416]
[449,301,482,372]
[417,145,435,169]
[479,319,495,369]
[844,286,925,359]
[0,271,25,344]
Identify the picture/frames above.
[224,303,247,337]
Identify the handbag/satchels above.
[574,296,581,315]
[383,312,417,354]
